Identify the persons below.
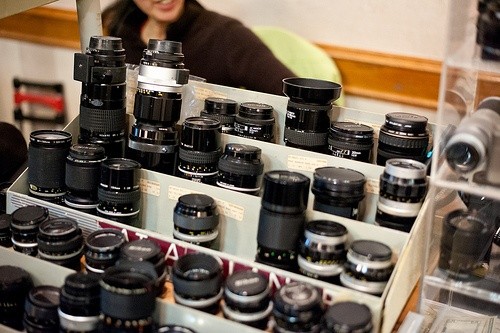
[101,0,298,97]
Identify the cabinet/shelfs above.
[0,68,447,333]
[418,0,500,332]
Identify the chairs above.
[248,26,344,107]
